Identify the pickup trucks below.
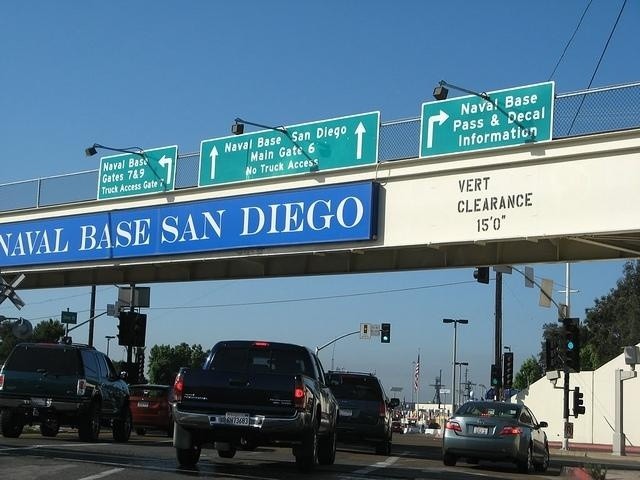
[171,338,341,472]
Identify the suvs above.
[0,343,134,441]
[324,369,400,455]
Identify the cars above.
[442,400,550,474]
[392,420,404,434]
[127,383,175,437]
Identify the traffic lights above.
[380,322,391,343]
[490,352,514,390]
[136,352,145,382]
[573,386,586,419]
[562,315,581,373]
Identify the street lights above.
[442,317,469,416]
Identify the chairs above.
[249,363,271,376]
